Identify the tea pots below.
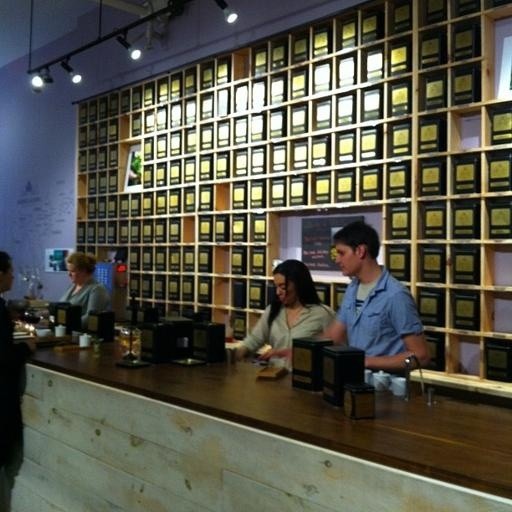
[20,263,45,299]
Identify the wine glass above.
[24,307,42,335]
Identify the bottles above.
[114,321,143,364]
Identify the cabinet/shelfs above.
[71,1,512,400]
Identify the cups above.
[54,323,66,339]
[79,333,91,350]
[363,367,407,397]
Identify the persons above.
[38,251,111,330]
[236,259,337,372]
[0,248,29,512]
[289,221,431,370]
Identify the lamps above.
[28,1,239,90]
